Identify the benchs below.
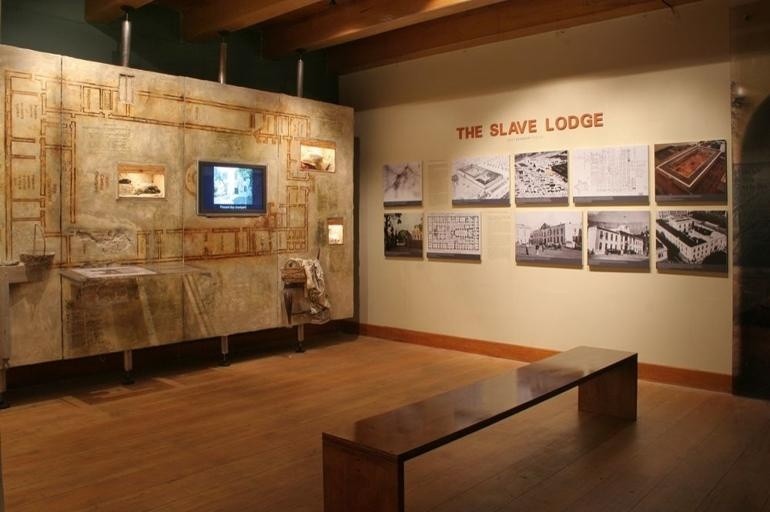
[322,346,638,512]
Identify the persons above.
[535,243,539,255]
[526,245,529,256]
[542,243,545,251]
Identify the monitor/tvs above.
[196,159,267,218]
